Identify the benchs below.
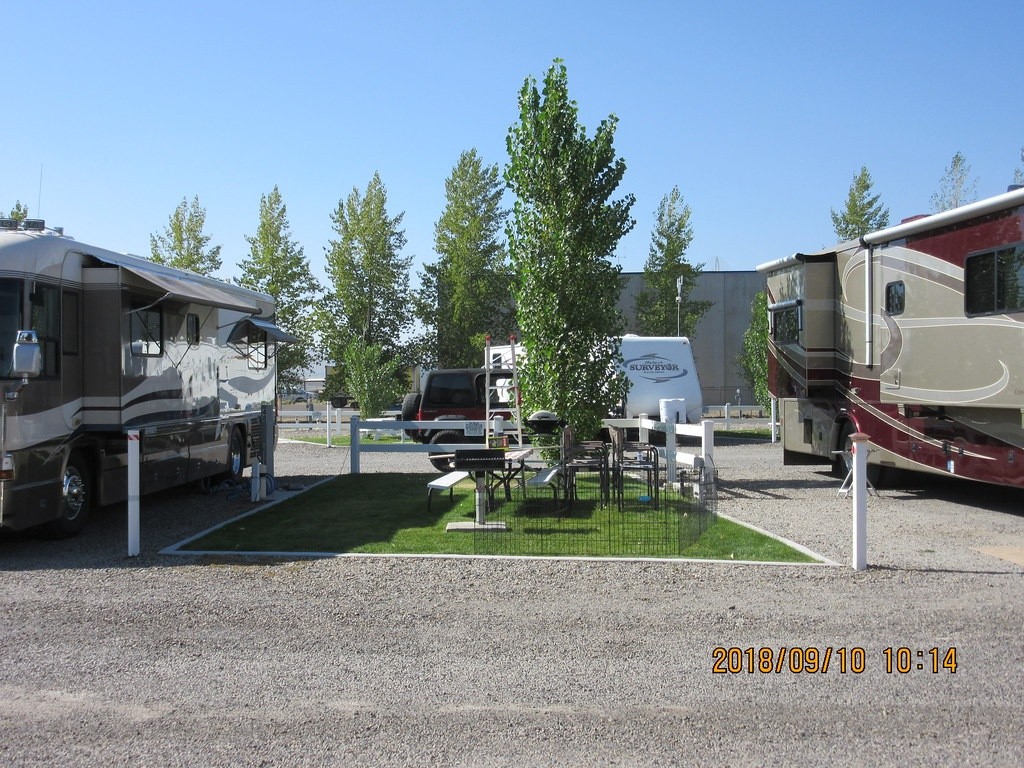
[524,462,569,512]
[426,471,474,511]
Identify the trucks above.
[1,215,301,540]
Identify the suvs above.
[395,367,528,474]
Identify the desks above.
[428,448,534,533]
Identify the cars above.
[281,386,312,404]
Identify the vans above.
[496,333,705,443]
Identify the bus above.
[755,183,1024,491]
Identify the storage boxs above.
[487,436,510,452]
